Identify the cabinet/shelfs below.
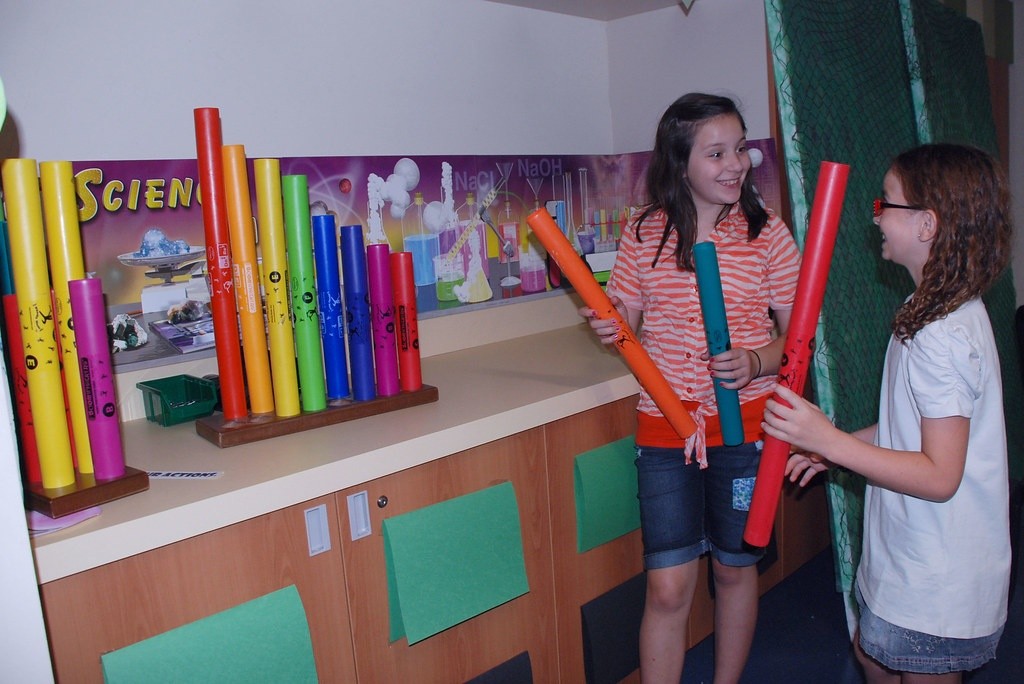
[27,316,829,684]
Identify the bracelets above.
[748,350,761,379]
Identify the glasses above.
[872,198,923,217]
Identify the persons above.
[578,93,815,684]
[761,143,1011,684]
